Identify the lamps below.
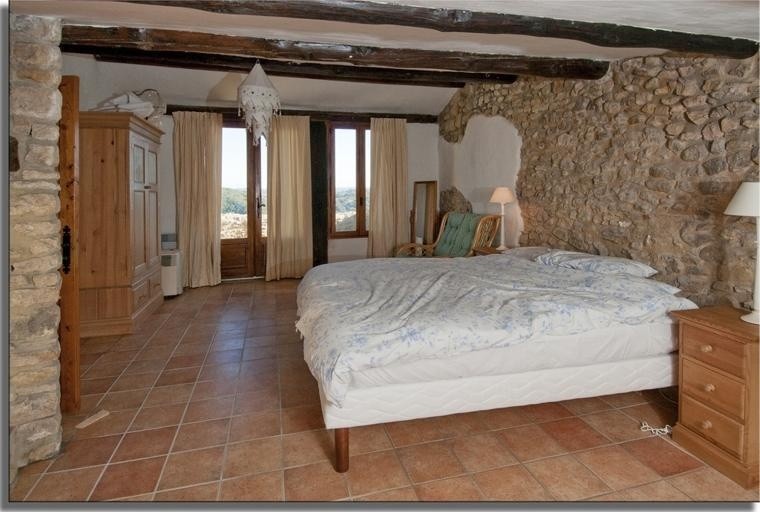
[489,186,515,250]
[236,56,284,147]
[720,179,760,325]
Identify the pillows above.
[505,244,659,277]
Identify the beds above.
[290,255,702,473]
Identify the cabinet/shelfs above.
[664,304,759,490]
[75,109,168,338]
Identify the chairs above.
[391,210,502,259]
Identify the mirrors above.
[410,181,437,252]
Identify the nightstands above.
[470,247,498,258]
